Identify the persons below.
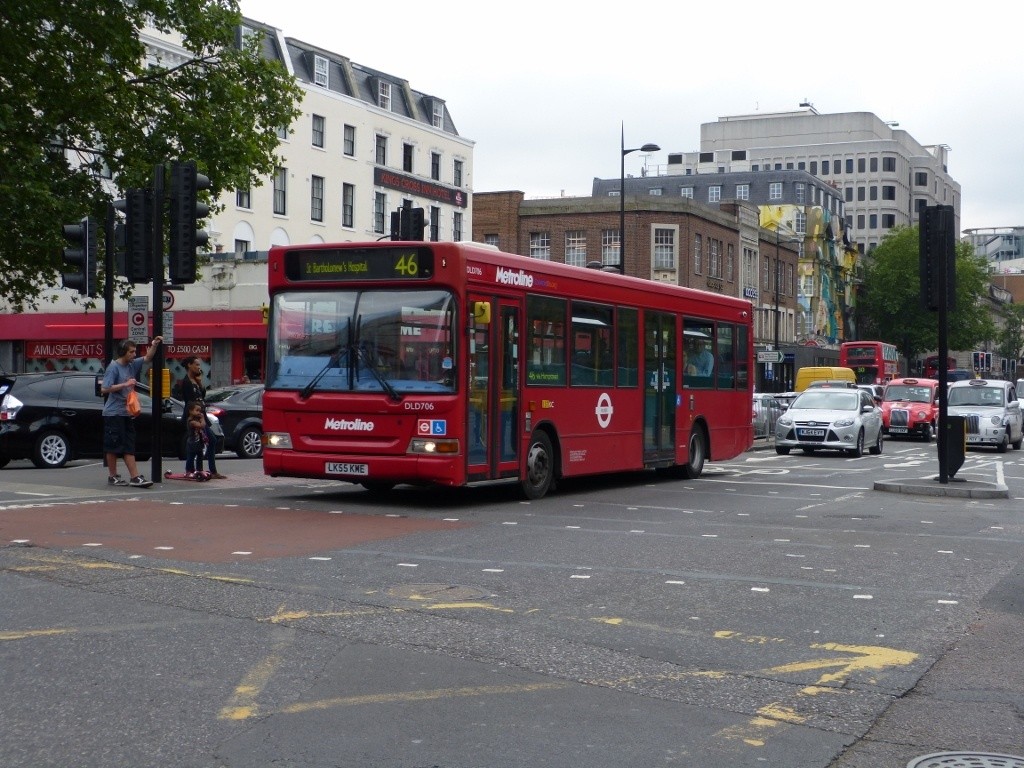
[242,376,250,384]
[181,356,227,479]
[101,336,163,487]
[683,336,714,377]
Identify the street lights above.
[584,122,661,276]
[752,226,803,352]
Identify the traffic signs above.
[756,351,786,365]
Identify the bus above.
[838,340,902,388]
[924,355,957,379]
[261,239,758,501]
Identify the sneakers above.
[107,475,128,485]
[129,475,153,487]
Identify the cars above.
[879,377,941,442]
[750,395,789,436]
[1013,378,1024,431]
[774,386,886,458]
[197,382,268,459]
[0,370,226,471]
[945,379,1023,454]
[809,380,859,389]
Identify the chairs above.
[572,350,735,388]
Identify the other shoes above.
[210,473,226,478]
[185,473,195,479]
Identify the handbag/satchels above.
[127,383,140,416]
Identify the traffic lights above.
[167,156,214,284]
[1010,359,1017,373]
[58,213,96,299]
[985,352,992,372]
[112,187,153,286]
[979,352,985,371]
[1000,359,1008,374]
[972,352,979,371]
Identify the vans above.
[792,365,859,393]
[947,369,977,384]
